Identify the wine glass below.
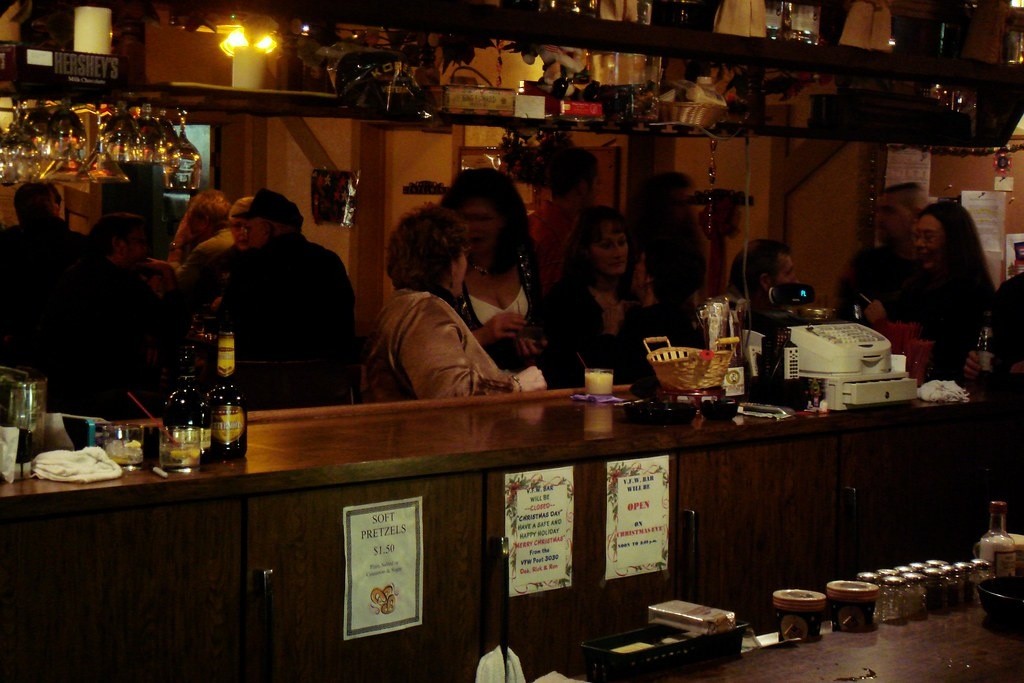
[80,91,202,188]
[0,93,86,183]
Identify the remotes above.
[739,402,795,415]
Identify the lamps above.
[208,15,278,60]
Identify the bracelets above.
[512,375,522,392]
[168,242,182,250]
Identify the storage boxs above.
[555,100,606,119]
[426,81,516,115]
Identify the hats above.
[231,188,304,228]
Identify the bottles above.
[894,566,928,620]
[977,312,995,371]
[826,580,879,632]
[697,77,717,130]
[205,332,247,460]
[954,559,992,602]
[980,501,1016,578]
[165,350,212,464]
[926,560,965,607]
[773,589,826,643]
[909,563,950,615]
[857,569,906,624]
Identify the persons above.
[444,167,549,383]
[548,172,706,383]
[713,239,800,328]
[362,203,547,402]
[525,148,596,299]
[839,181,1024,390]
[0,181,187,420]
[167,189,357,366]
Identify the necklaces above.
[473,263,489,274]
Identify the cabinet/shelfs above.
[0,408,1024,683]
[0,0,1024,150]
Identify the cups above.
[518,327,542,347]
[585,369,613,395]
[232,46,266,91]
[103,425,144,465]
[74,7,112,54]
[160,425,201,470]
[592,53,661,121]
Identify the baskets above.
[642,336,740,390]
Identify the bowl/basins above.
[1006,533,1024,575]
[977,577,1024,629]
